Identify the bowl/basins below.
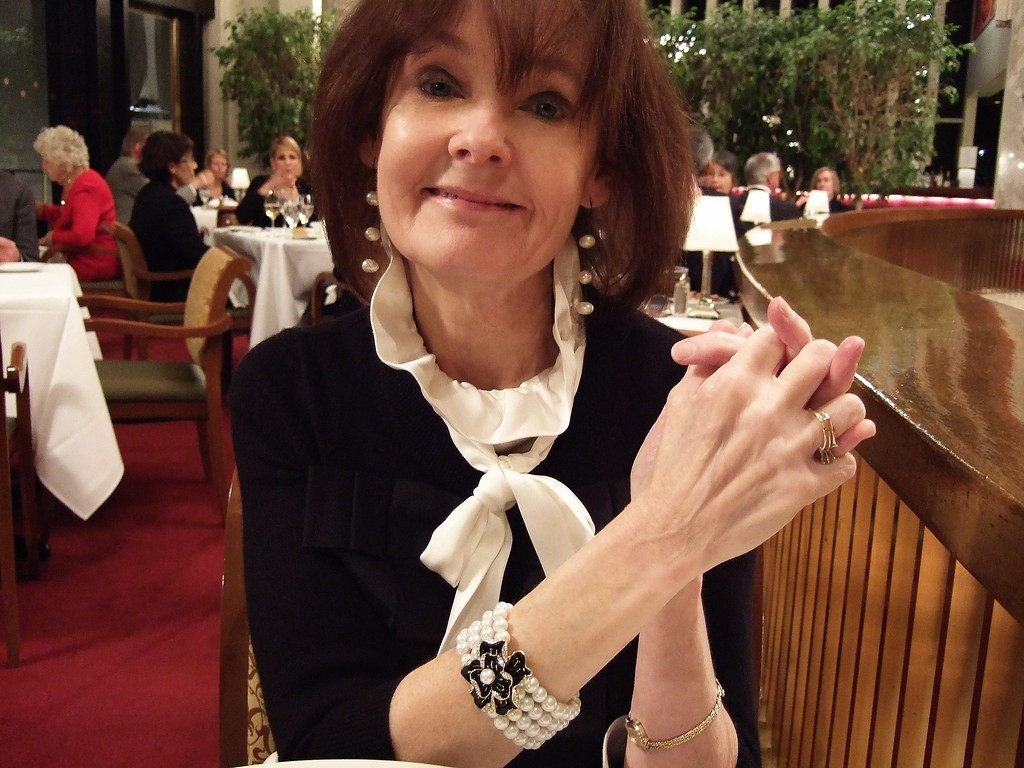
[663,266,688,295]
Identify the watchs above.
[623,677,725,751]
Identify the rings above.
[809,407,839,465]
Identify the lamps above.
[230,165,250,201]
[681,197,739,318]
[802,190,830,215]
[740,190,772,226]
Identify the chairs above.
[0,158,276,768]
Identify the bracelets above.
[456,601,582,750]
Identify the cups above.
[199,186,209,208]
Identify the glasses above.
[178,157,195,166]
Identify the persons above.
[678,125,855,295]
[105,125,319,304]
[0,164,39,262]
[31,126,121,282]
[228,0,878,768]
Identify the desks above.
[213,225,336,348]
[0,262,125,521]
[644,291,741,341]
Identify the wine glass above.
[264,188,314,240]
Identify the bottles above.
[675,275,714,311]
